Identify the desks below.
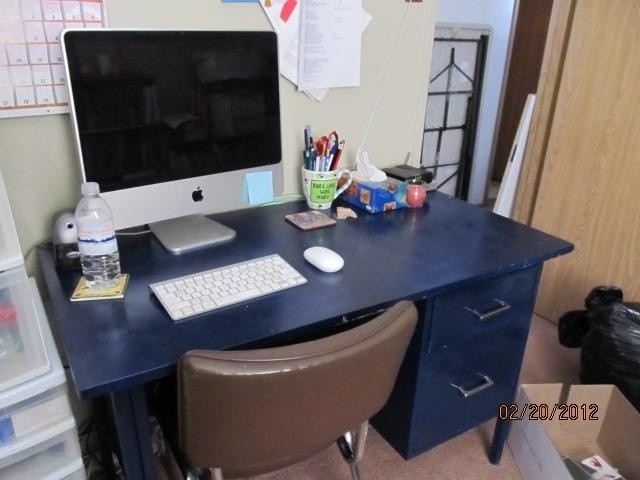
[35,187,576,479]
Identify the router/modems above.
[380,162,433,185]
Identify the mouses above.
[304,246,345,273]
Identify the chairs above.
[175,300,420,480]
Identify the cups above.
[302,166,353,211]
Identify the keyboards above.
[149,253,307,321]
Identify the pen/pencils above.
[302,128,345,173]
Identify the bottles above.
[75,183,120,290]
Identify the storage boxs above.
[507,383,640,480]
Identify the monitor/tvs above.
[61,29,283,254]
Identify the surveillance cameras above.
[53,214,83,272]
